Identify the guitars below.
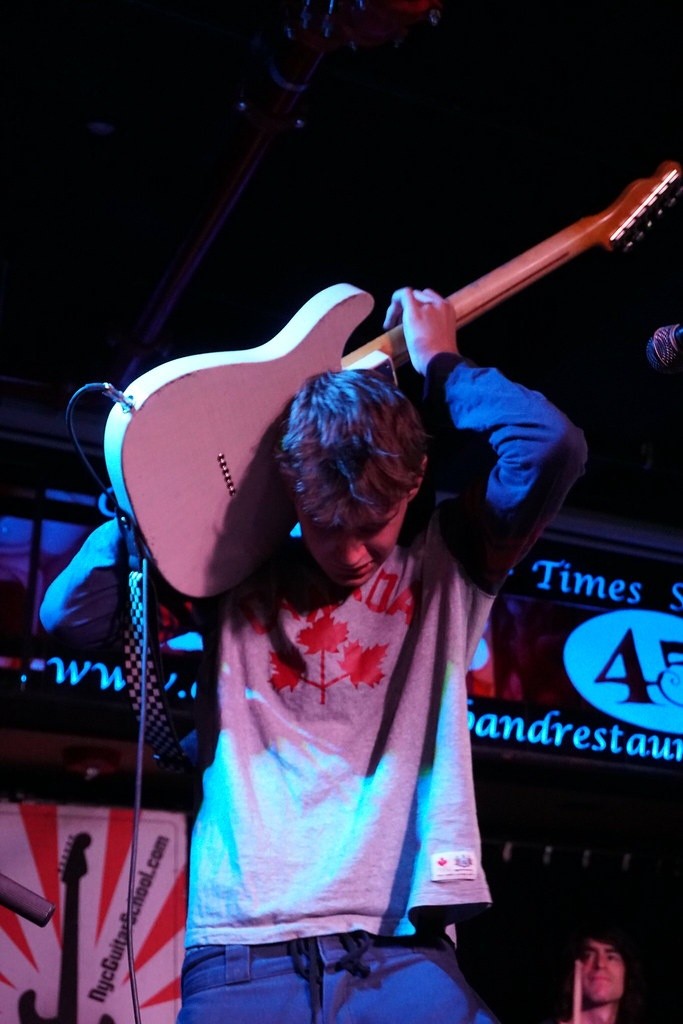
[103,160,683,597]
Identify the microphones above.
[647,323,683,371]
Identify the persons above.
[558,923,628,1024]
[40,288,586,1024]
